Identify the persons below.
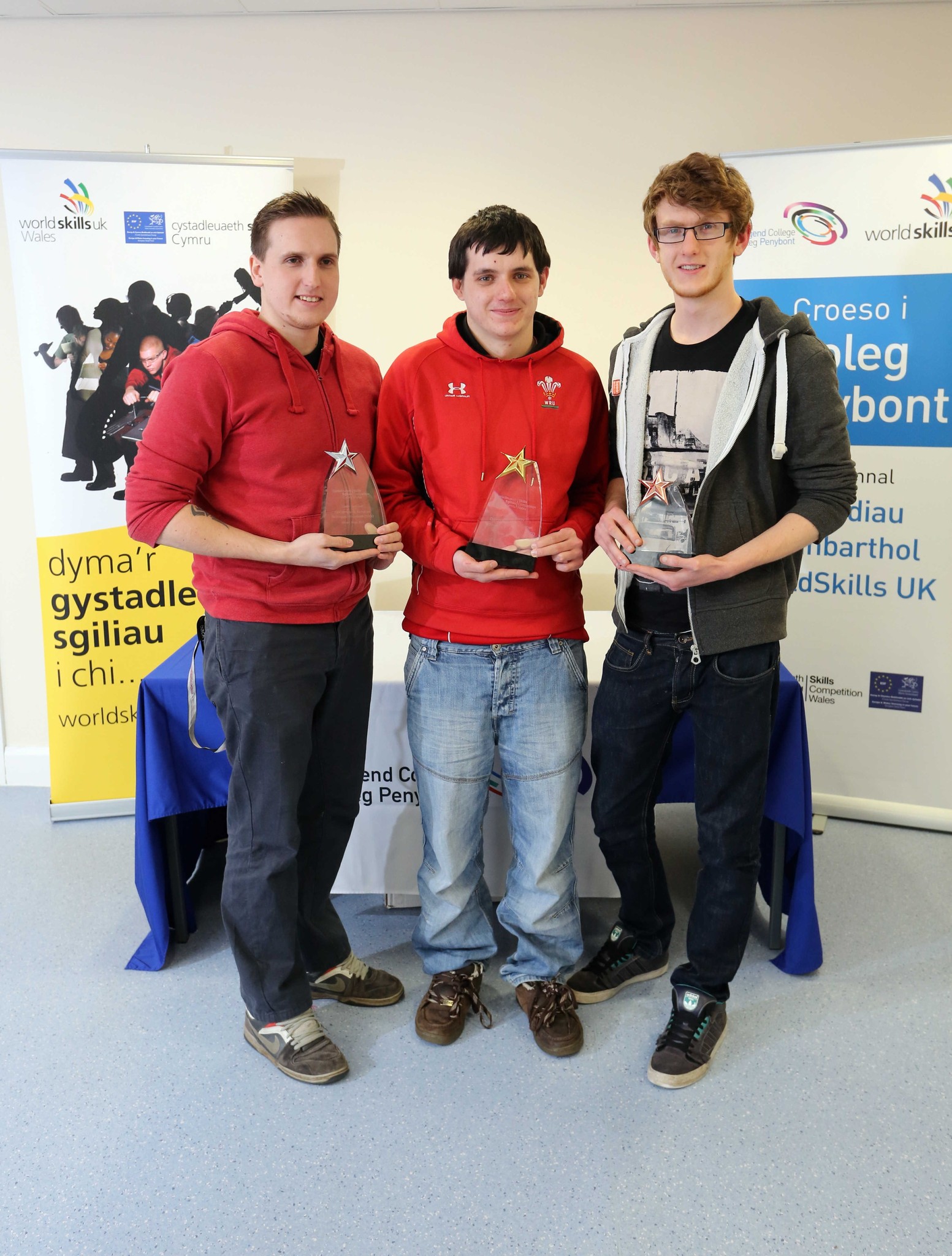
[39,281,233,501]
[372,204,613,1060]
[574,150,854,1082]
[126,194,404,1084]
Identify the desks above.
[124,610,826,977]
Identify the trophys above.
[623,468,696,573]
[464,447,542,573]
[320,438,387,551]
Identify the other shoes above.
[86,476,116,490]
[113,490,125,500]
[61,469,93,481]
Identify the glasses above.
[653,222,733,244]
[139,350,164,364]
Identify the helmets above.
[166,293,191,316]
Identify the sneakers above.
[515,972,584,1057]
[564,921,669,1004]
[309,950,404,1006]
[415,962,492,1046]
[648,985,728,1089]
[244,1005,349,1084]
